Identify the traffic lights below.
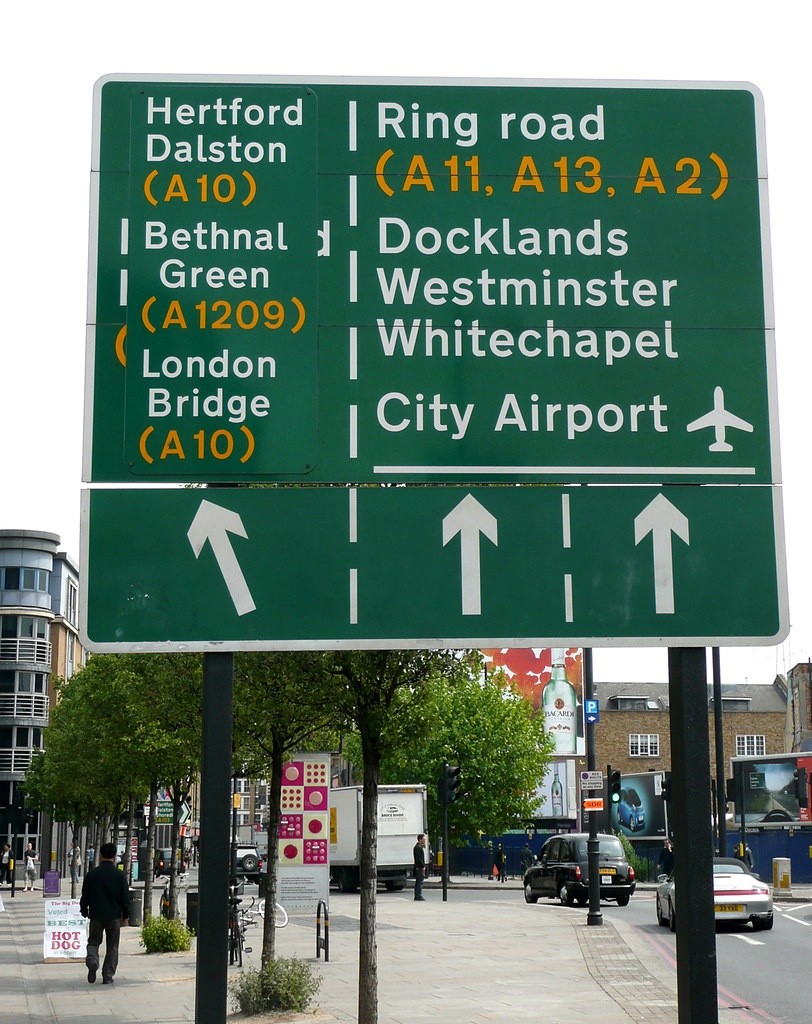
[608,769,622,803]
[661,771,672,803]
[444,766,461,804]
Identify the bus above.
[110,826,144,881]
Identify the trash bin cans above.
[186,893,198,937]
[122,889,143,927]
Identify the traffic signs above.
[146,800,192,825]
[78,73,792,649]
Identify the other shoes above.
[23,888,27,891]
[31,888,33,891]
[415,897,425,901]
[103,979,114,984]
[88,960,98,983]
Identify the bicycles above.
[229,878,289,967]
[158,872,190,921]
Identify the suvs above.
[230,841,264,883]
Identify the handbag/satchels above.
[76,856,81,866]
[493,864,500,876]
[27,859,34,870]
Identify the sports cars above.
[656,856,774,932]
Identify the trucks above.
[330,783,431,894]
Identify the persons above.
[89,844,95,870]
[495,844,507,882]
[79,843,131,984]
[413,834,426,901]
[68,843,81,883]
[0,843,13,887]
[22,843,38,892]
[735,843,754,868]
[185,846,193,853]
[656,838,673,878]
[521,842,533,869]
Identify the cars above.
[524,832,637,907]
[154,847,187,878]
[617,786,646,832]
